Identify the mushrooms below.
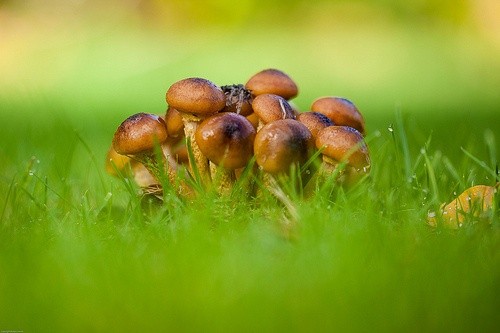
[106,68,372,204]
[426,184,495,228]
